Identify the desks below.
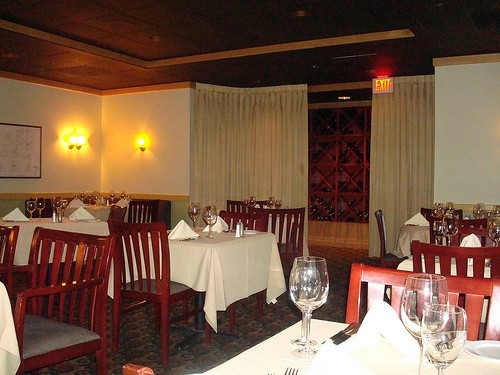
[202,319,500,375]
[224,215,309,261]
[386,256,491,323]
[107,227,286,333]
[0,218,110,266]
[0,282,21,375]
[391,224,461,261]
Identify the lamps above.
[137,137,147,151]
[68,135,85,150]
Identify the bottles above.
[309,108,369,223]
[234,221,244,237]
[58,211,62,223]
[52,211,56,223]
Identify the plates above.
[463,340,500,363]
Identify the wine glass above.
[288,255,329,358]
[202,204,217,238]
[400,274,449,375]
[188,201,201,232]
[420,304,467,375]
[432,201,500,266]
[26,197,68,223]
[243,196,282,212]
[75,191,125,206]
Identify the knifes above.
[321,322,360,345]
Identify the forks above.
[284,368,298,375]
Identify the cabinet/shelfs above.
[307,109,370,225]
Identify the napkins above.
[404,212,429,226]
[202,216,228,233]
[168,220,199,239]
[69,206,95,220]
[313,301,430,370]
[459,233,482,265]
[2,207,29,221]
[69,198,84,208]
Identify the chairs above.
[0,199,500,375]
